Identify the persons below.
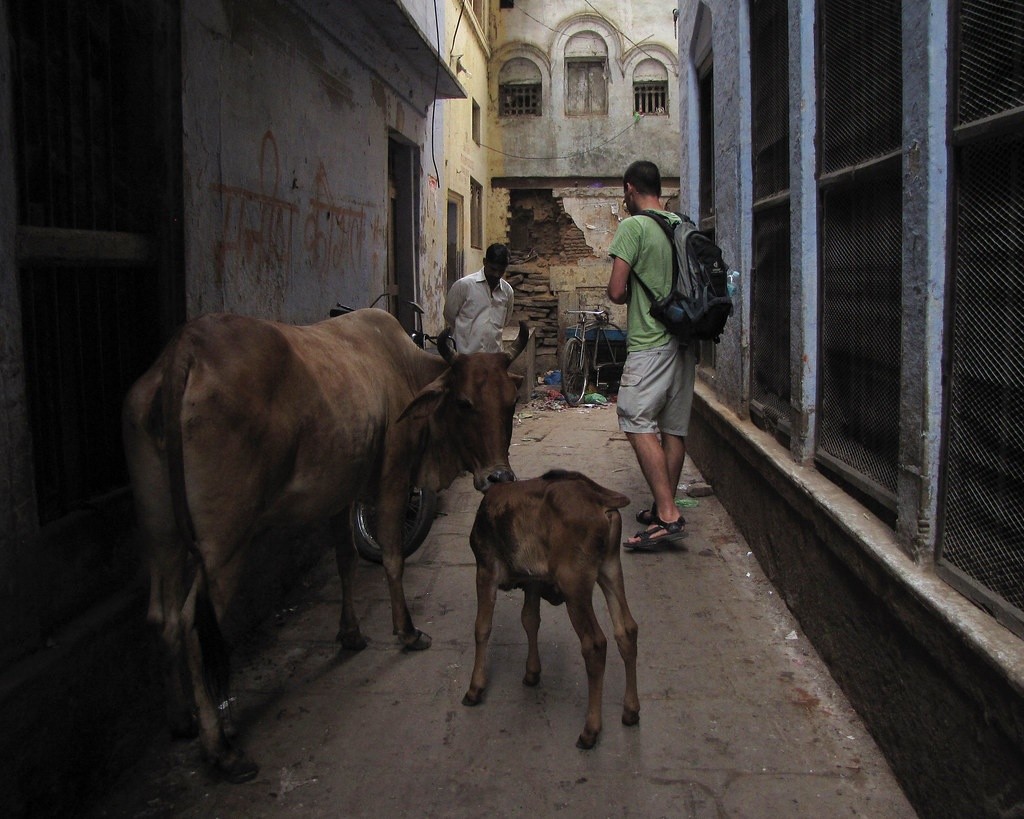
[444,243,514,353]
[607,160,697,548]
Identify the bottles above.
[726,271,741,300]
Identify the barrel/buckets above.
[545,372,561,385]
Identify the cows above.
[127,307,641,783]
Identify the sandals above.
[636,502,658,526]
[623,515,689,548]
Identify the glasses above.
[621,198,629,213]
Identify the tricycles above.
[562,308,628,407]
[329,289,456,563]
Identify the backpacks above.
[631,210,733,351]
